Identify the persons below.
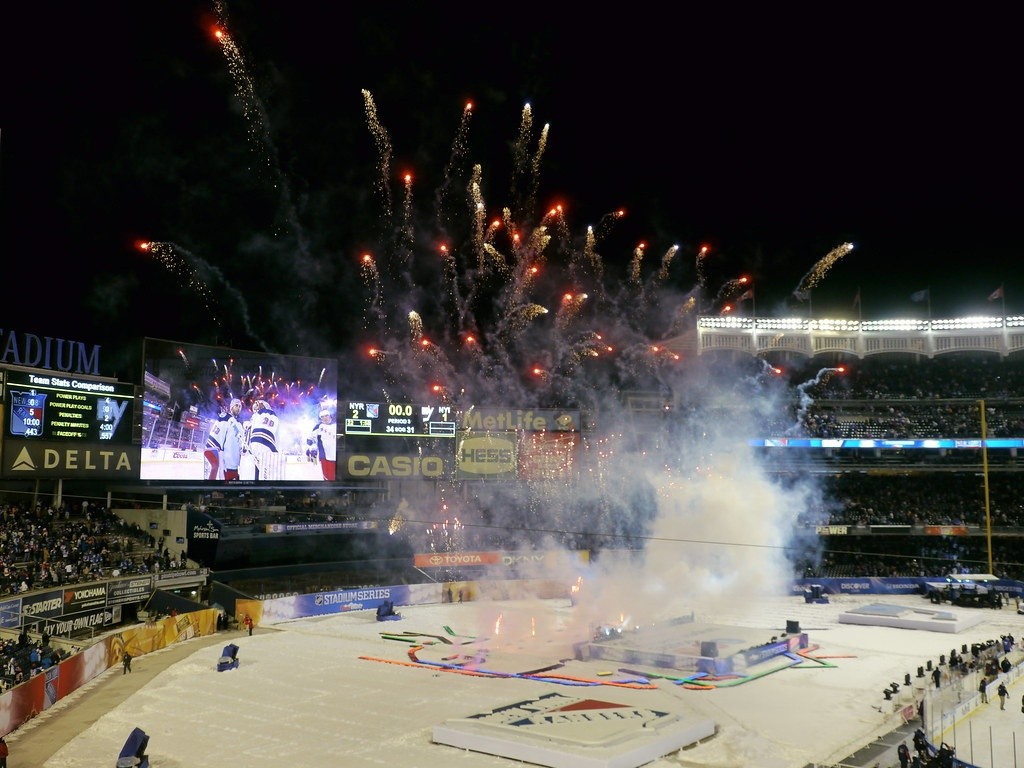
[405,501,657,555]
[979,678,989,705]
[204,399,246,480]
[306,409,336,480]
[922,581,1024,617]
[985,632,1015,679]
[217,613,229,631]
[897,700,934,768]
[243,401,279,480]
[998,682,1010,710]
[770,474,1024,527]
[750,371,1024,439]
[195,493,402,525]
[0,499,190,600]
[0,736,8,768]
[244,614,254,636]
[932,666,941,691]
[447,588,464,603]
[783,537,1024,577]
[0,628,71,696]
[123,652,131,674]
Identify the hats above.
[318,409,331,418]
[230,398,242,409]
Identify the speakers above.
[701,642,719,657]
[786,620,801,633]
